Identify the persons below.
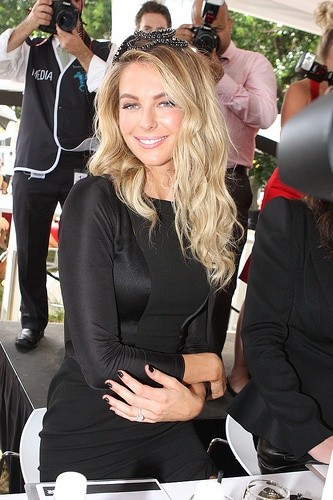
[136,0,172,32]
[226,192,333,475]
[176,0,278,355]
[226,0,333,394]
[0,0,118,349]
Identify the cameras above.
[187,0,225,57]
[38,0,78,34]
[295,51,333,88]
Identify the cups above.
[243,479,290,500]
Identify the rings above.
[38,28,245,482]
[137,408,144,422]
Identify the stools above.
[207,413,261,476]
[0,407,47,483]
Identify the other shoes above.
[226,375,238,399]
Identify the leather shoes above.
[15,328,44,353]
